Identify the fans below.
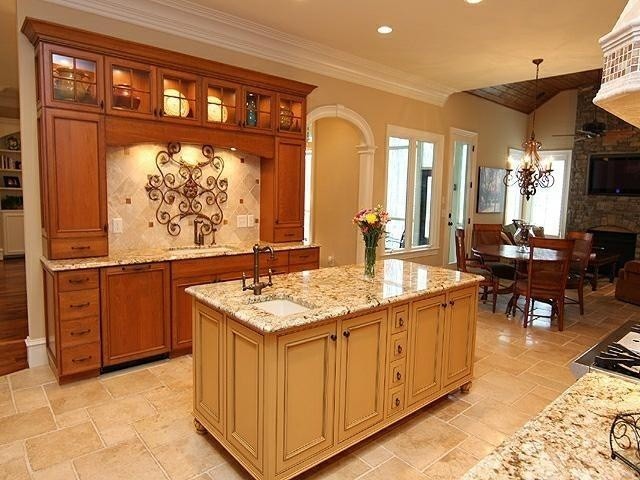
[551,104,638,144]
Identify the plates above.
[5,135,21,150]
[163,89,190,118]
[207,95,228,123]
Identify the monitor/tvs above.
[586,153,640,197]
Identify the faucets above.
[194,217,205,246]
[253,244,275,294]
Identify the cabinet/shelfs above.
[190,282,478,479]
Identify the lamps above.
[503,59,555,202]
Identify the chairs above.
[454,223,593,332]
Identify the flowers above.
[352,205,392,276]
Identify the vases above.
[362,247,376,282]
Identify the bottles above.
[248,93,257,126]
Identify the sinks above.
[248,294,312,318]
[167,246,233,255]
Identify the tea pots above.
[53,66,94,103]
